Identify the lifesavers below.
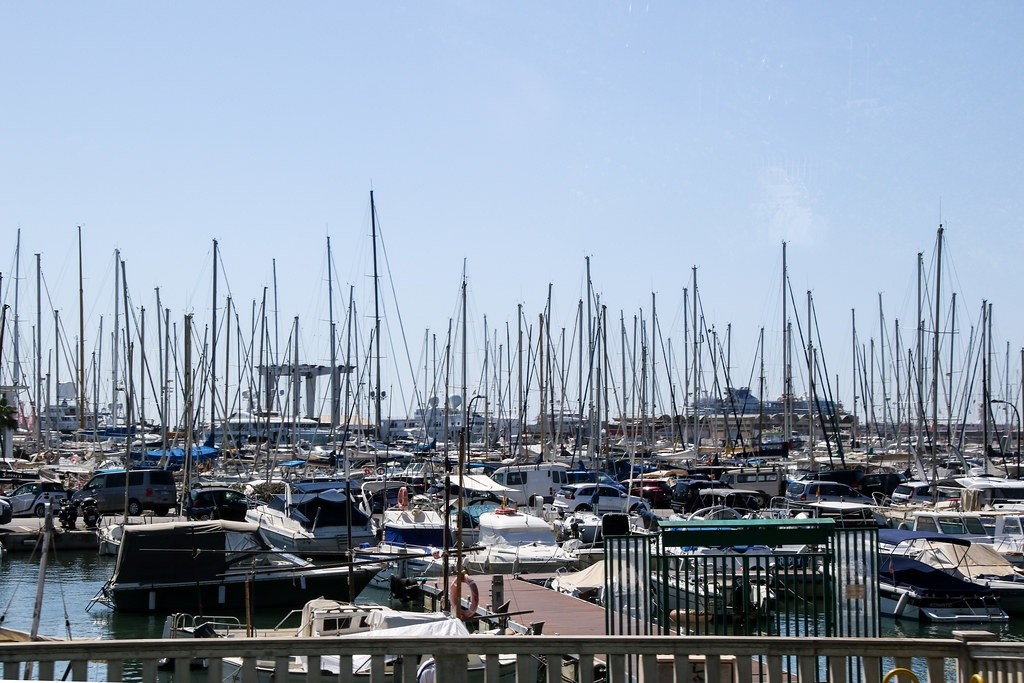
[398,487,409,510]
[377,466,386,475]
[494,508,516,515]
[364,468,370,477]
[449,573,480,618]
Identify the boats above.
[0,187,1022,683]
